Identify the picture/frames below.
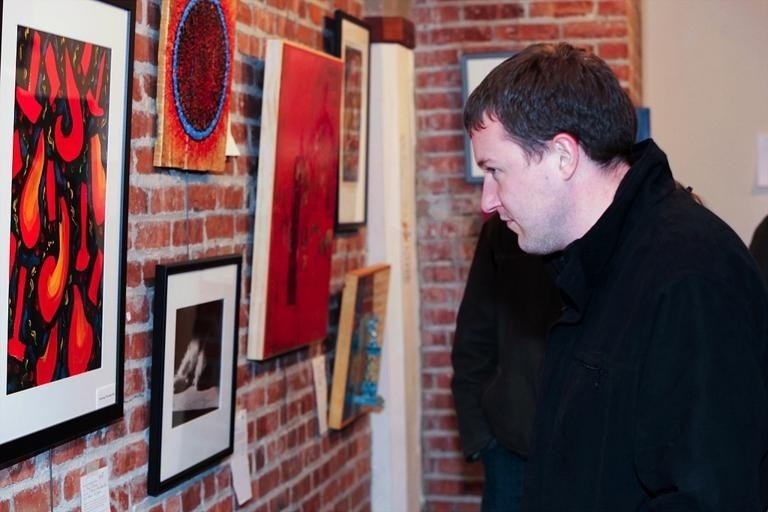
[0,2,141,468]
[459,52,517,185]
[333,8,369,231]
[145,253,242,497]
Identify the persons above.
[462,41,767,512]
